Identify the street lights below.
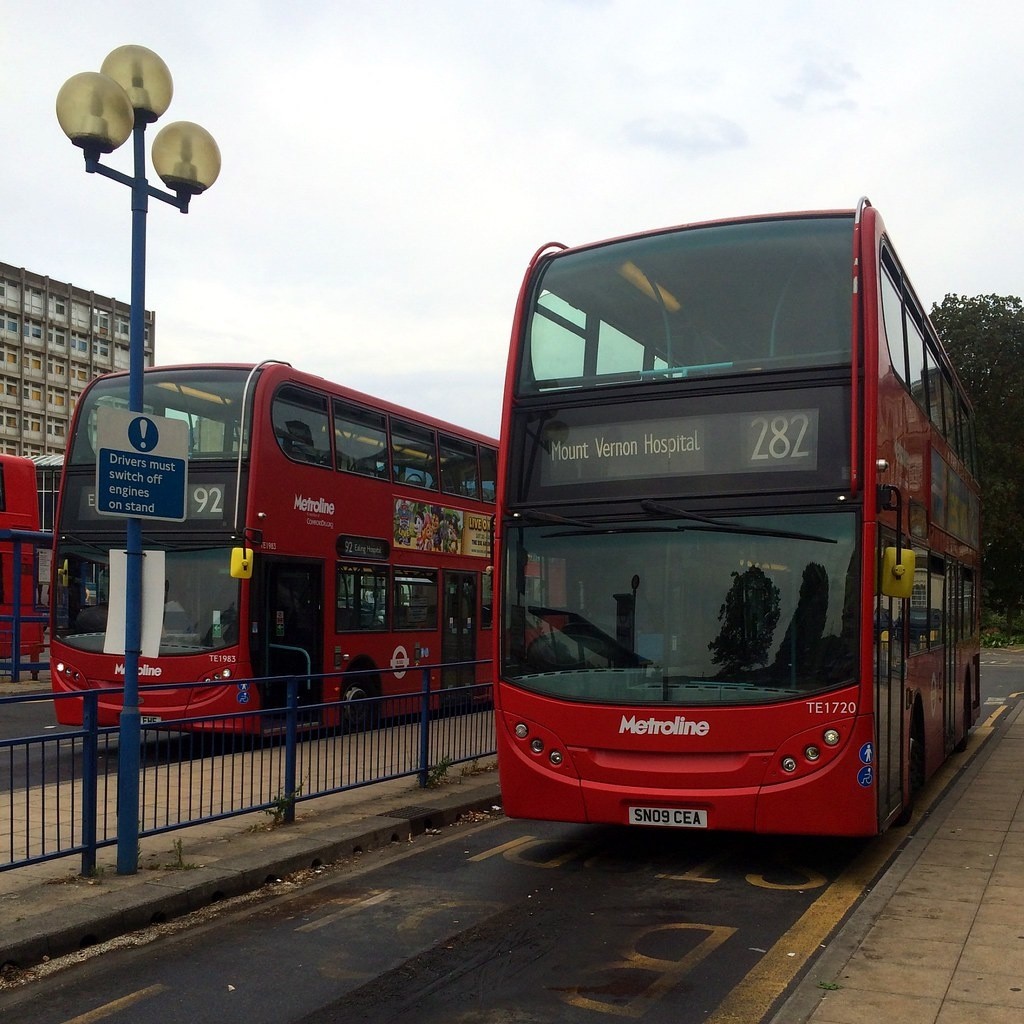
[54,43,224,876]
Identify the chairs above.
[320,448,448,491]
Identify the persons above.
[84,587,90,605]
[365,588,374,612]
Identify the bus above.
[483,195,986,847]
[45,360,569,741]
[0,452,45,663]
